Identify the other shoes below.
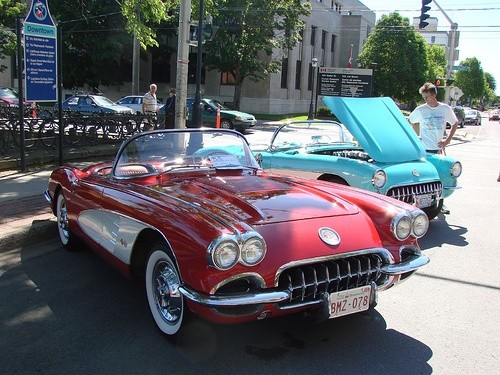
[440,208,450,213]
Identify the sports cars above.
[43,127,431,344]
[190,95,463,221]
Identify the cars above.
[446,106,465,129]
[114,95,167,116]
[157,97,258,133]
[0,86,33,119]
[464,107,500,126]
[53,94,135,124]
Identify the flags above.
[348,47,353,68]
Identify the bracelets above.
[445,139,450,144]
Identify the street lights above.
[307,57,318,120]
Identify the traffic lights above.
[418,0,432,28]
[435,78,446,88]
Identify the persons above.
[142,84,160,139]
[479,104,483,111]
[407,82,458,155]
[492,100,497,107]
[165,88,177,129]
